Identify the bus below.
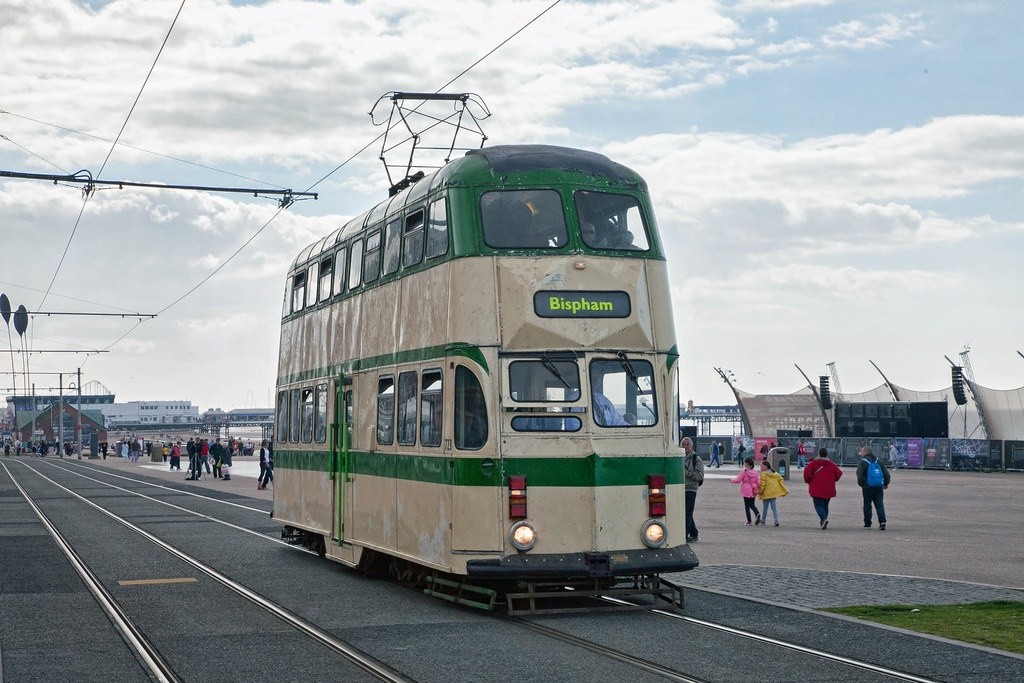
[270,91,701,618]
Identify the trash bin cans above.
[152,443,163,462]
[768,447,790,480]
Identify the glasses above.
[583,229,594,234]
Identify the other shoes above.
[879,522,885,530]
[686,535,699,542]
[863,524,871,529]
[743,521,751,526]
[258,484,268,490]
[820,520,828,529]
[754,514,760,525]
[774,521,779,526]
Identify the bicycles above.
[948,455,974,472]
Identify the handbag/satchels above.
[209,457,216,465]
[220,464,230,475]
[217,459,222,469]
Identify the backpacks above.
[863,457,884,488]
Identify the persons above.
[162,441,182,470]
[562,372,631,431]
[221,442,232,480]
[228,437,243,456]
[122,438,141,463]
[706,440,719,469]
[622,414,637,426]
[22,439,45,458]
[726,441,900,529]
[617,230,642,250]
[557,197,621,247]
[581,222,595,243]
[55,439,60,455]
[101,439,108,460]
[258,436,273,489]
[681,437,704,542]
[64,440,78,456]
[146,441,152,457]
[187,437,224,480]
[5,438,21,458]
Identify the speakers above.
[820,376,832,409]
[952,367,967,405]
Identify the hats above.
[680,437,693,449]
